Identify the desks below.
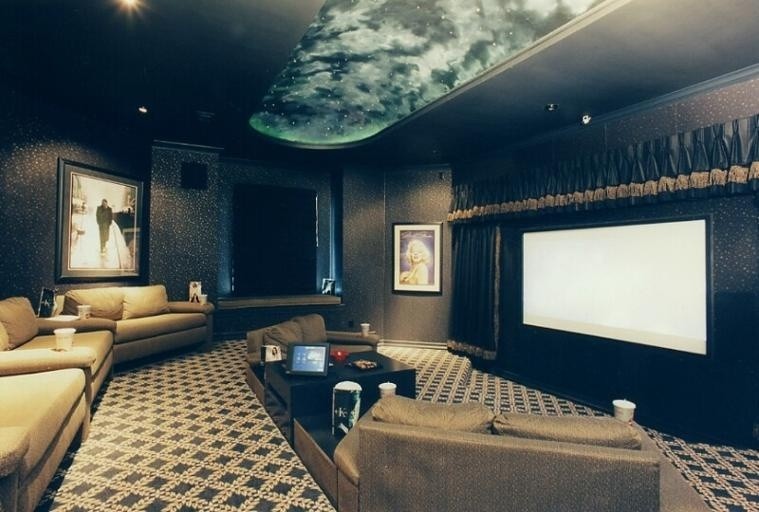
[214,294,341,311]
[265,351,416,448]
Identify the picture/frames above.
[54,156,150,285]
[391,222,443,296]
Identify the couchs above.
[0,296,116,512]
[54,285,215,364]
[246,321,379,406]
[334,402,715,512]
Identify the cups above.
[330,348,346,361]
[76,305,90,320]
[612,399,636,425]
[359,323,370,336]
[198,295,208,305]
[54,328,76,350]
[378,383,397,398]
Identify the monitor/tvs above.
[520,213,716,358]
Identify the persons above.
[401,238,433,284]
[190,281,200,304]
[268,347,282,361]
[95,198,114,255]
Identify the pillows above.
[291,313,327,344]
[493,413,641,450]
[0,296,39,351]
[264,321,303,359]
[122,285,170,320]
[60,287,123,321]
[372,393,494,436]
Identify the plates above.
[346,362,382,370]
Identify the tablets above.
[286,341,330,376]
